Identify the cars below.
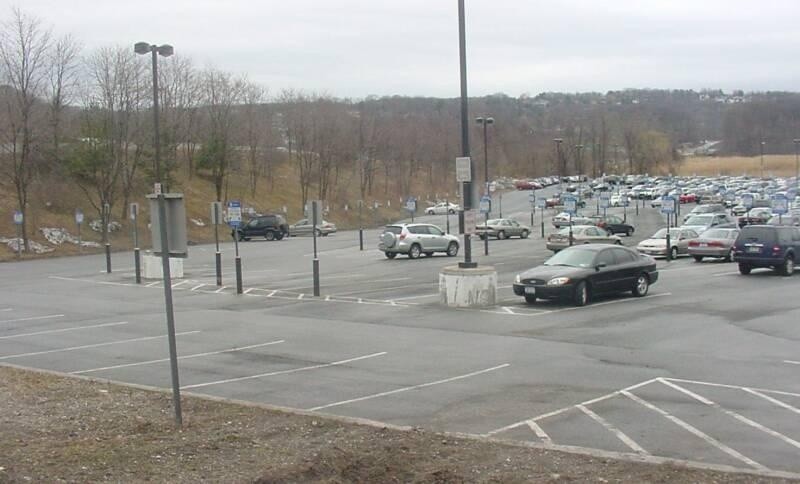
[475,217,532,240]
[283,217,337,236]
[424,201,459,214]
[513,242,658,306]
[672,139,720,158]
[688,227,741,262]
[545,224,624,254]
[636,227,699,259]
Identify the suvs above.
[230,214,289,241]
[378,222,460,258]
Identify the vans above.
[732,224,800,276]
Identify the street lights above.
[596,142,602,174]
[575,143,584,187]
[554,137,563,191]
[611,143,618,172]
[475,115,494,255]
[760,136,767,178]
[792,137,800,182]
[135,41,182,431]
